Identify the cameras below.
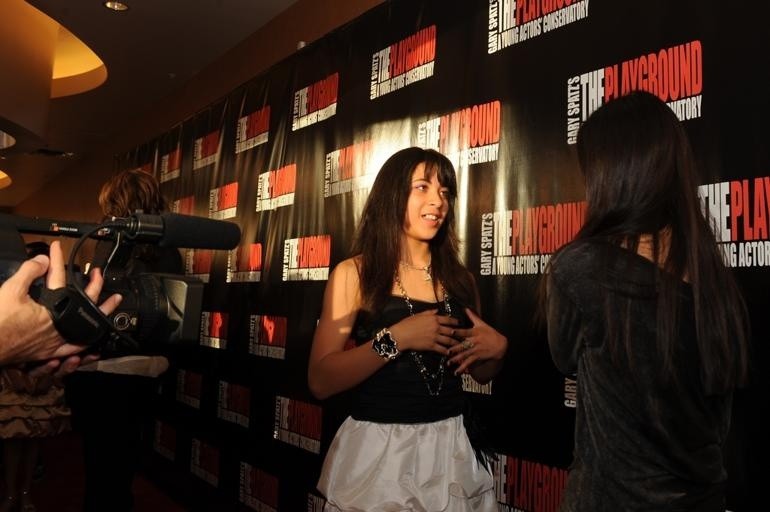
[1,214,183,357]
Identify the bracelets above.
[372,327,399,361]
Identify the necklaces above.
[392,268,451,398]
[390,252,433,282]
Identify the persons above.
[540,90,742,512]
[307,148,507,512]
[93,169,188,308]
[1,239,122,376]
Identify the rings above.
[461,339,474,350]
[451,330,457,337]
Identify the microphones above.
[127,213,240,250]
